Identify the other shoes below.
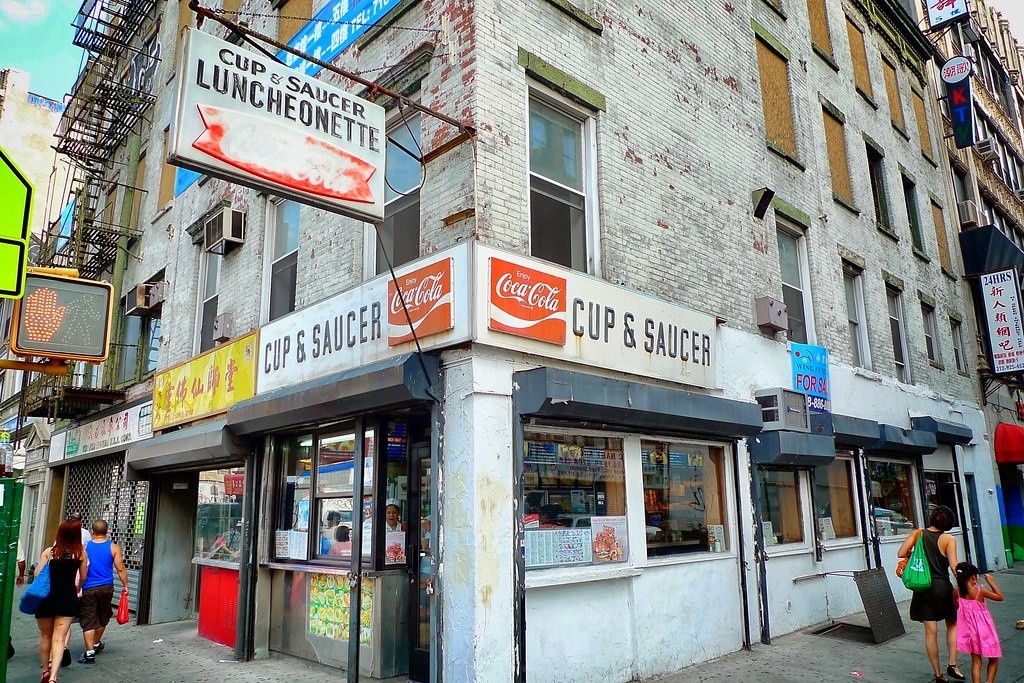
[947,664,966,681]
[8,636,17,660]
[934,673,948,683]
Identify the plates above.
[310,576,372,644]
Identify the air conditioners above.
[202,206,245,257]
[125,283,162,319]
[975,137,1002,161]
[959,200,990,228]
[756,389,811,432]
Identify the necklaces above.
[93,536,104,539]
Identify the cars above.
[558,514,662,544]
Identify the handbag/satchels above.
[19,547,53,615]
[902,532,932,590]
[896,527,925,577]
[117,594,129,624]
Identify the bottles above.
[883,528,888,536]
[714,539,721,553]
[821,529,828,540]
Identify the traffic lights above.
[10,272,114,360]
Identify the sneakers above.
[78,652,95,664]
[94,641,105,654]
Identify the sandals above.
[40,670,51,683]
[40,661,52,670]
[48,680,56,683]
[60,649,71,666]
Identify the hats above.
[386,498,401,508]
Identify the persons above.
[34,519,87,683]
[8,513,92,668]
[386,498,402,531]
[421,515,431,548]
[210,518,241,562]
[539,504,569,527]
[897,505,965,683]
[80,519,130,663]
[320,512,351,556]
[952,562,1004,683]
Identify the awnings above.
[994,423,1024,464]
